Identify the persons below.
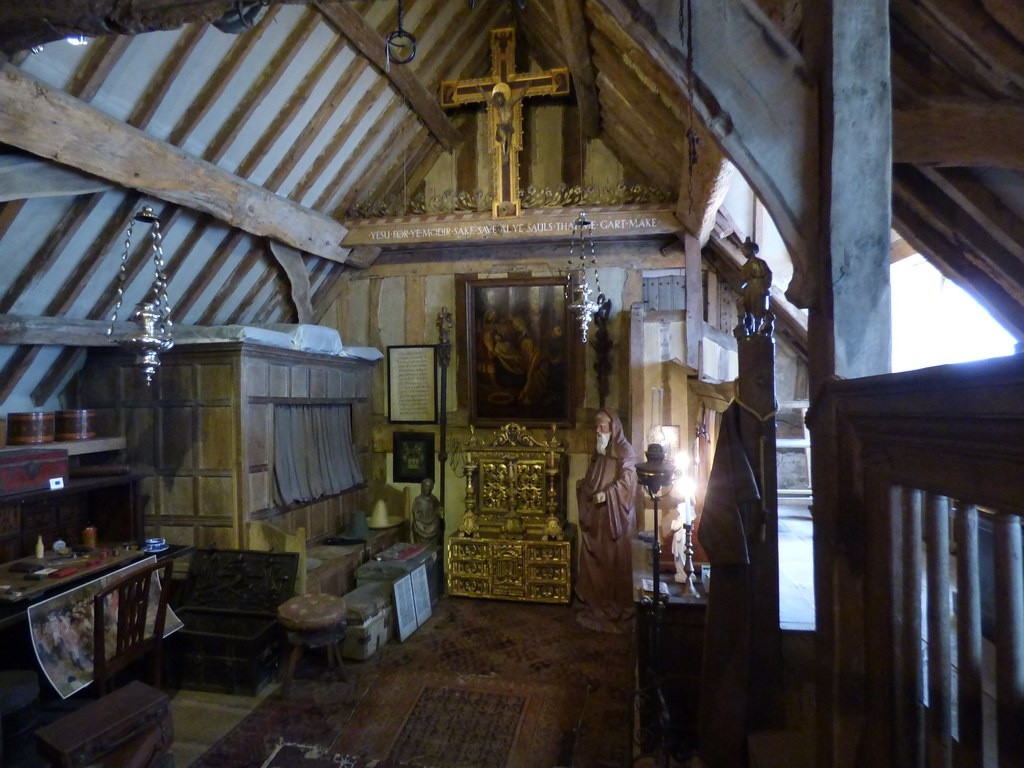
[737,237,772,334]
[475,81,533,163]
[410,479,445,546]
[574,412,641,621]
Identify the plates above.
[140,544,169,554]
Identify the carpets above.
[386,683,531,768]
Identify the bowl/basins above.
[6,411,55,446]
[54,409,98,441]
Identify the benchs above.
[266,481,410,591]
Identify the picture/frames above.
[392,431,436,483]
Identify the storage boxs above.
[355,541,443,626]
[336,581,400,660]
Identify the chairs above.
[41,554,173,703]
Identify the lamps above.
[107,205,176,382]
[634,444,681,714]
[564,80,603,342]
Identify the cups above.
[82,527,98,548]
[145,537,165,551]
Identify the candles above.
[348,505,368,537]
[684,480,691,526]
[370,495,388,526]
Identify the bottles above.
[35,534,45,558]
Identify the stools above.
[280,543,440,681]
